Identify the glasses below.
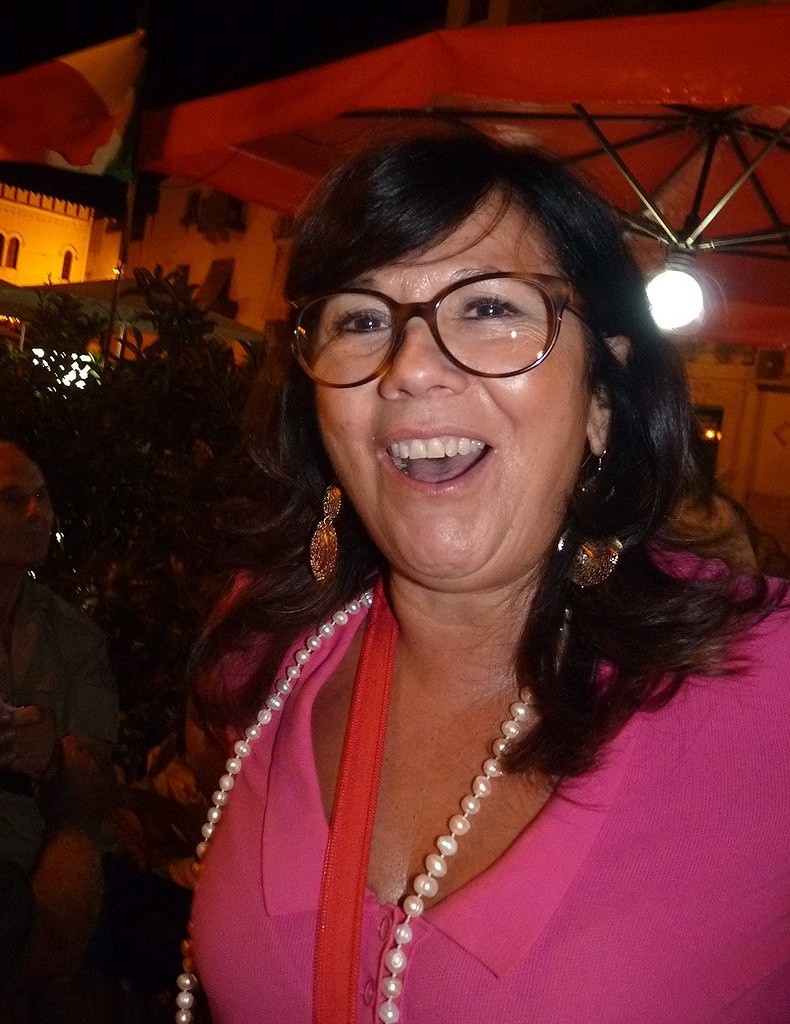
[286,271,591,388]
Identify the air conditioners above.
[753,348,790,392]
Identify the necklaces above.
[177,590,573,1024]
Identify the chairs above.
[0,815,105,1024]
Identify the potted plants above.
[178,189,247,243]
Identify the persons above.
[0,442,116,1024]
[176,129,790,1024]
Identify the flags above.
[0,28,144,177]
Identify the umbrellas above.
[160,0,789,351]
